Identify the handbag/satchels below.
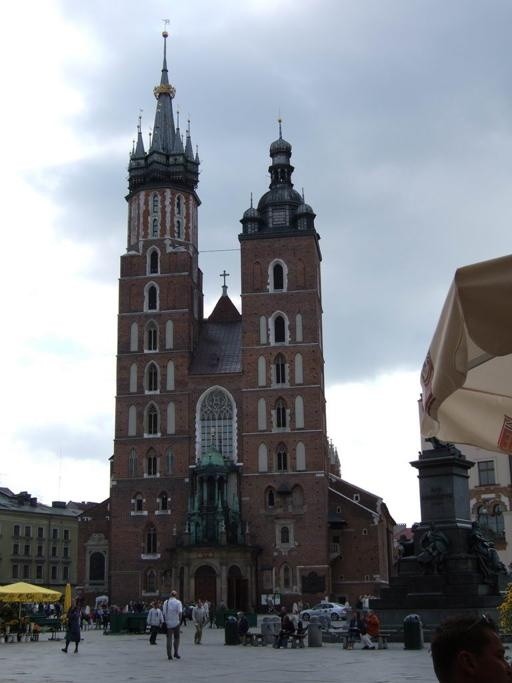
[226,616,237,623]
[361,623,366,635]
[160,622,167,633]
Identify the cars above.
[298,600,352,622]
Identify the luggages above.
[225,623,240,645]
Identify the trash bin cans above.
[403,614,424,650]
[308,615,322,647]
[260,617,281,644]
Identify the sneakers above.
[62,649,67,652]
[74,650,78,653]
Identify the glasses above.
[465,614,490,632]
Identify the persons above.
[162,590,181,661]
[471,519,509,575]
[416,521,450,577]
[0,598,381,651]
[429,612,512,682]
[60,599,80,653]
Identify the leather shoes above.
[168,657,172,659]
[362,646,375,649]
[174,654,180,659]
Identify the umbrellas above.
[419,252,511,459]
[0,581,63,618]
[60,582,73,623]
